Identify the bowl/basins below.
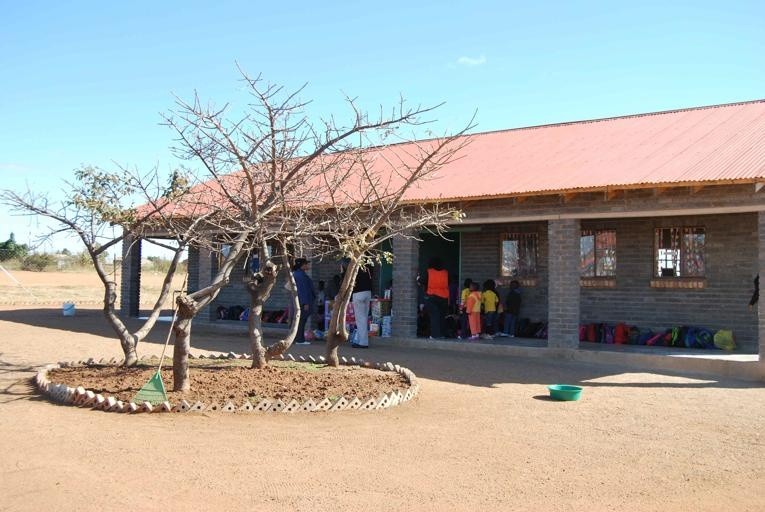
[547,384,583,401]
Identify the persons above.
[748,272,758,312]
[293,257,523,349]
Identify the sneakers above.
[457,332,513,340]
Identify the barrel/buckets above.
[63,303,75,317]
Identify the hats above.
[295,258,311,266]
